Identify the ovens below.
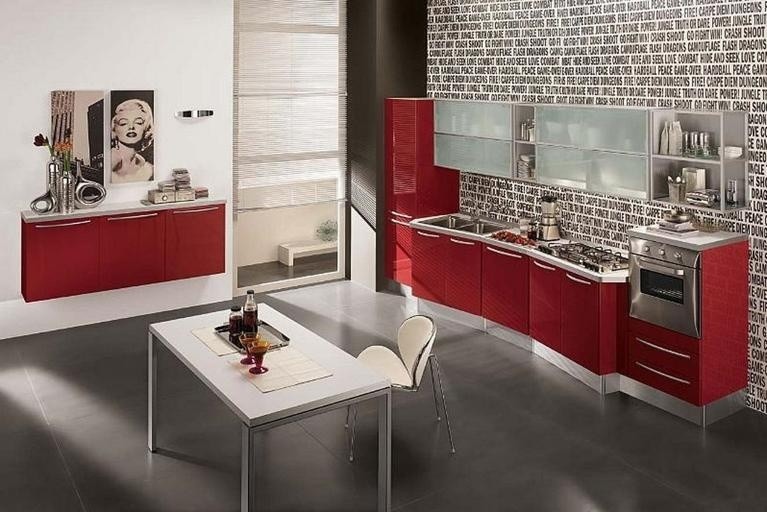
[627,254,700,339]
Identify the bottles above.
[243,290,258,333]
[680,131,710,147]
[521,117,534,143]
[528,221,537,239]
[228,306,243,334]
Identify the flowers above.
[33,127,74,167]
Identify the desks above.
[149,302,392,512]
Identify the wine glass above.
[239,334,269,373]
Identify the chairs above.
[344,316,456,462]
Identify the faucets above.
[460,196,479,217]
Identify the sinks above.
[411,214,475,229]
[456,219,513,236]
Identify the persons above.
[111,98,154,183]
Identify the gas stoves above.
[538,242,630,272]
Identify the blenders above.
[537,195,562,241]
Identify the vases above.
[58,169,74,213]
[47,157,63,199]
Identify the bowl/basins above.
[663,211,692,221]
[716,146,742,159]
[521,153,536,162]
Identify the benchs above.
[277,240,338,268]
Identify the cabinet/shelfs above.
[528,258,619,377]
[651,106,749,213]
[383,97,459,287]
[513,104,651,206]
[482,244,528,335]
[165,204,225,282]
[21,211,101,303]
[435,96,514,183]
[410,227,482,318]
[101,208,165,292]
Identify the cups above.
[519,218,530,236]
